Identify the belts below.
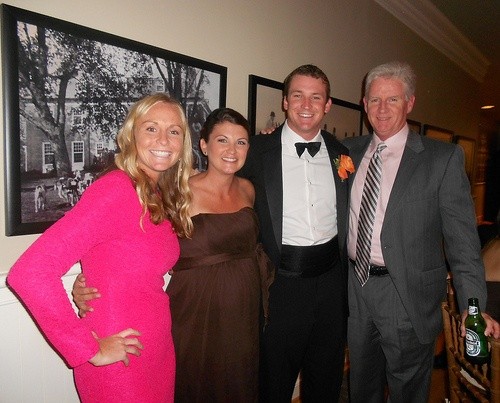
[347,256,389,275]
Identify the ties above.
[353,142,387,288]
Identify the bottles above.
[464,297,490,364]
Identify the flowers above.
[334,154,356,183]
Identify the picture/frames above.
[406,117,479,196]
[0,3,227,238]
[247,73,364,145]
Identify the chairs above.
[439,273,500,403]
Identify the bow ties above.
[295,142,321,159]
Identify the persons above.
[72,107,275,403]
[261,62,500,403]
[189,65,356,403]
[6,95,192,403]
